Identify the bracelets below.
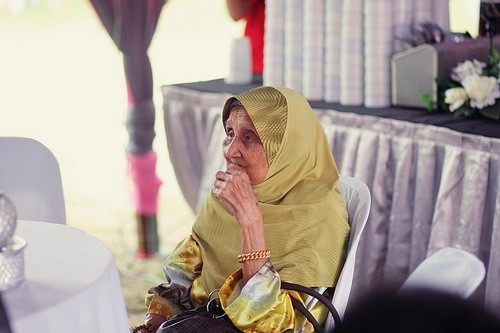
[237,248,271,262]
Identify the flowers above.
[421,47,500,119]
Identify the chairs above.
[324,174,486,332]
[0,137,66,223]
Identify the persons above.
[128,84,351,332]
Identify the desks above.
[2,219,131,333]
[162,78,500,318]
